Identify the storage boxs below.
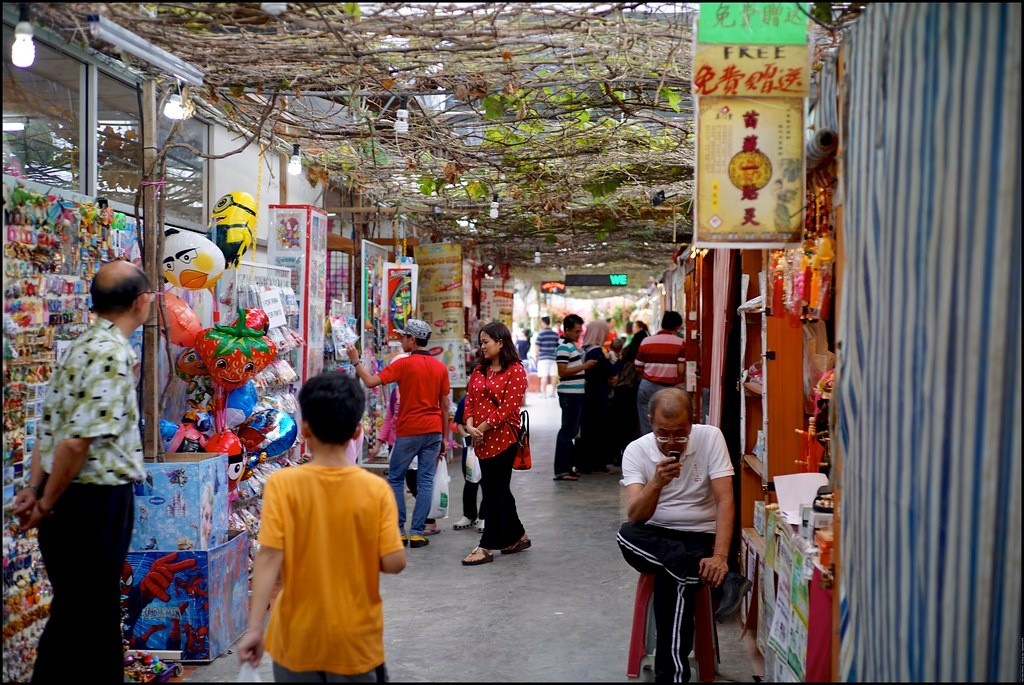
[114,450,261,666]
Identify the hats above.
[392,318,433,340]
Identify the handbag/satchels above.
[512,409,532,470]
[465,445,481,484]
[426,456,451,520]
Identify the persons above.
[615,384,754,682]
[236,369,408,682]
[370,354,450,537]
[346,318,451,549]
[513,305,703,486]
[452,361,487,534]
[4,256,157,684]
[460,322,533,567]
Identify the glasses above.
[137,290,156,302]
[654,436,691,443]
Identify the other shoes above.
[714,574,753,624]
[402,534,429,548]
[453,517,484,533]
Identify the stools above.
[625,568,723,685]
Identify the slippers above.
[461,547,494,566]
[553,474,577,482]
[501,539,532,554]
[423,528,441,536]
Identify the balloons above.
[141,187,299,494]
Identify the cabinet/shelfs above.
[724,231,861,682]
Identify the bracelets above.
[24,483,39,491]
[352,360,363,368]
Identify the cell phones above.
[668,451,681,464]
